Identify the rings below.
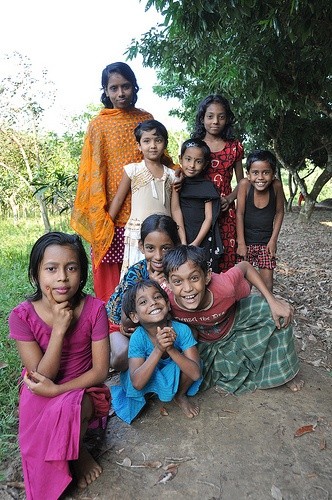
[27,386,30,390]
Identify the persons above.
[159,243,306,398]
[8,229,111,500]
[236,150,283,296]
[106,120,187,282]
[188,94,245,273]
[71,61,186,333]
[108,279,204,423]
[298,180,307,205]
[179,138,226,273]
[103,214,184,372]
